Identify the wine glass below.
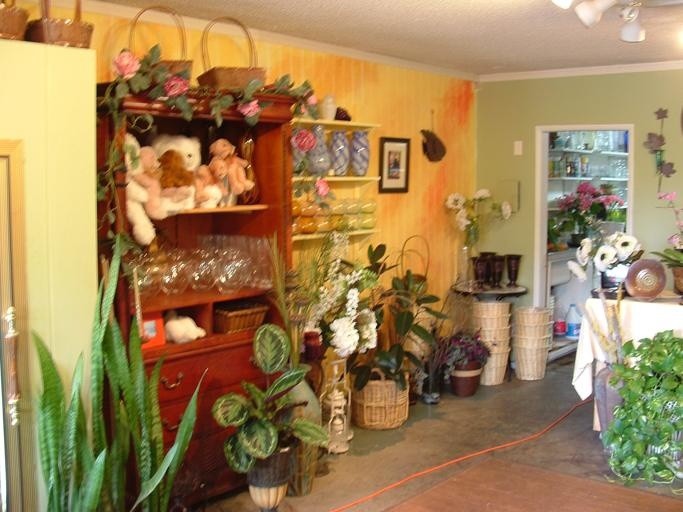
[468,252,523,292]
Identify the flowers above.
[441,189,513,242]
[437,328,491,373]
[100,47,321,124]
[563,228,647,283]
[308,230,382,359]
[547,183,623,247]
[645,191,683,267]
[643,102,677,193]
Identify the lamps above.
[547,0,681,45]
[322,373,353,454]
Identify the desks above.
[446,278,528,383]
[570,292,682,448]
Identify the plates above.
[623,258,665,302]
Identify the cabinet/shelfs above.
[92,78,312,511]
[547,248,604,366]
[286,117,383,241]
[546,147,627,213]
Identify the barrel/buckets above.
[566,303,582,341]
[554,301,566,335]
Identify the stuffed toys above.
[124,133,256,247]
[165,314,207,346]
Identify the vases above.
[462,243,481,286]
[449,362,483,398]
[669,262,682,298]
[288,124,373,178]
[599,253,643,297]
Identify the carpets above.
[381,454,681,512]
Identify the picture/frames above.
[376,135,412,194]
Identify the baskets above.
[472,297,556,387]
[351,366,411,432]
[0,0,29,41]
[27,1,98,51]
[193,14,267,99]
[122,5,192,79]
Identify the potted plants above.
[250,208,347,497]
[598,325,682,488]
[209,316,329,511]
[350,242,445,429]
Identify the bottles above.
[292,199,377,236]
[293,128,371,178]
[136,244,258,297]
[547,133,627,204]
[320,94,336,120]
